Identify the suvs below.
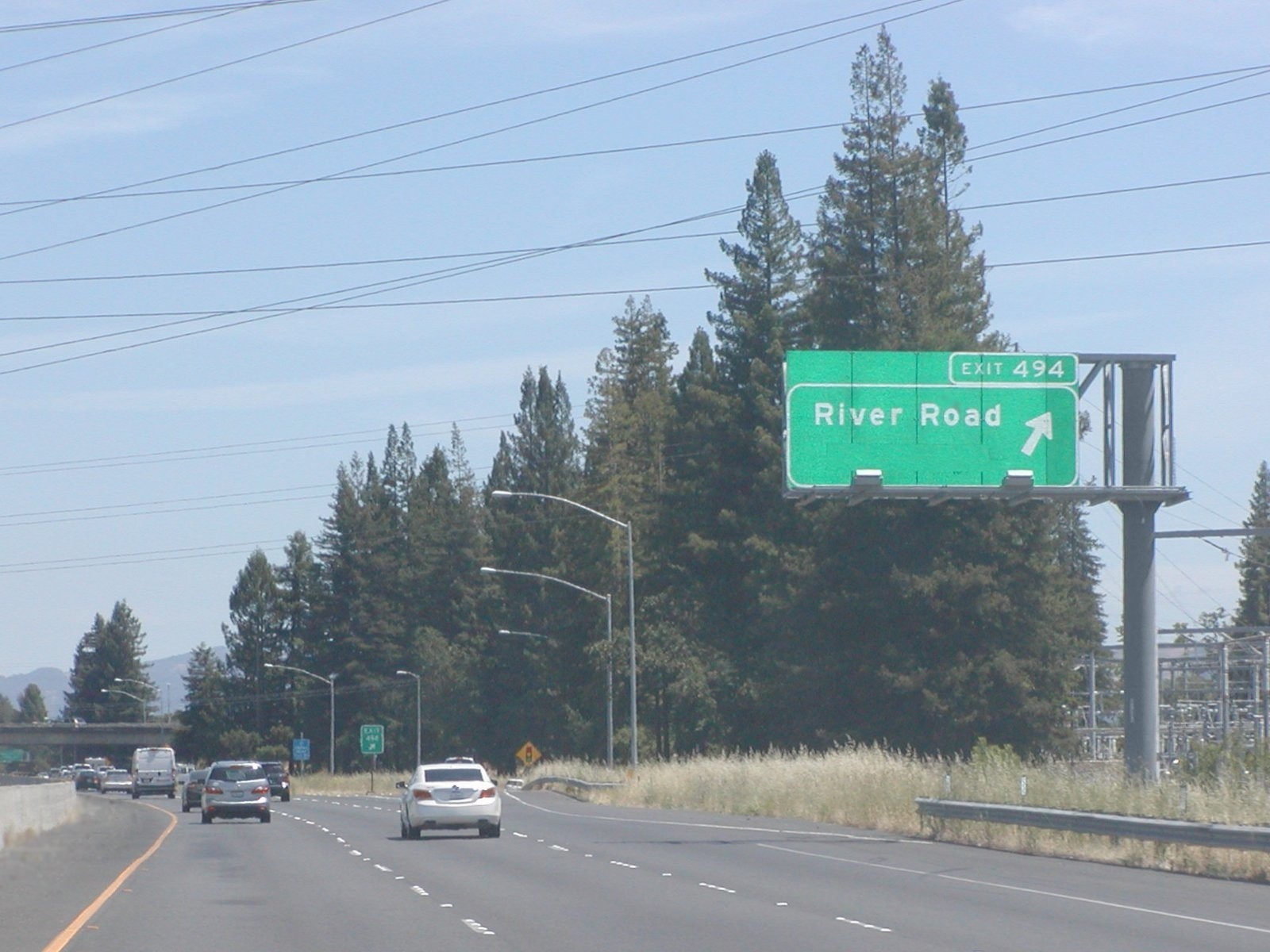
[197,760,271,823]
[260,762,291,801]
[51,716,86,728]
[445,756,473,763]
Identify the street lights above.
[115,678,165,748]
[395,671,421,766]
[493,488,639,766]
[480,565,613,763]
[264,663,334,775]
[101,689,146,724]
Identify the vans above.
[131,748,176,798]
[176,764,194,783]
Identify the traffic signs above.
[784,351,1080,489]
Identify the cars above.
[100,769,132,794]
[183,769,208,812]
[76,769,99,790]
[395,764,502,837]
[38,758,113,779]
